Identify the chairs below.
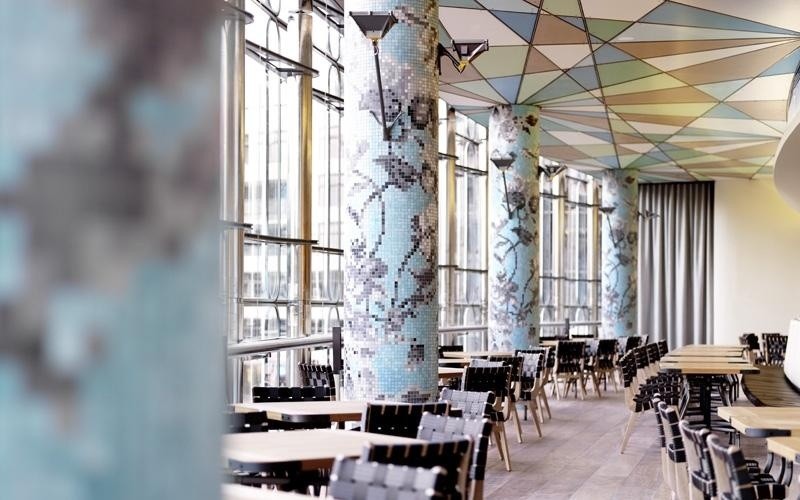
[539,334,650,401]
[740,332,788,367]
[619,340,685,454]
[224,387,494,500]
[439,345,553,471]
[299,363,337,402]
[652,393,786,500]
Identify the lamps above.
[487,155,516,221]
[636,208,662,222]
[598,206,619,250]
[347,8,406,143]
[435,37,490,77]
[537,163,569,182]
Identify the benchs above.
[742,367,800,408]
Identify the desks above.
[662,341,760,427]
[716,407,799,447]
[767,437,800,489]
